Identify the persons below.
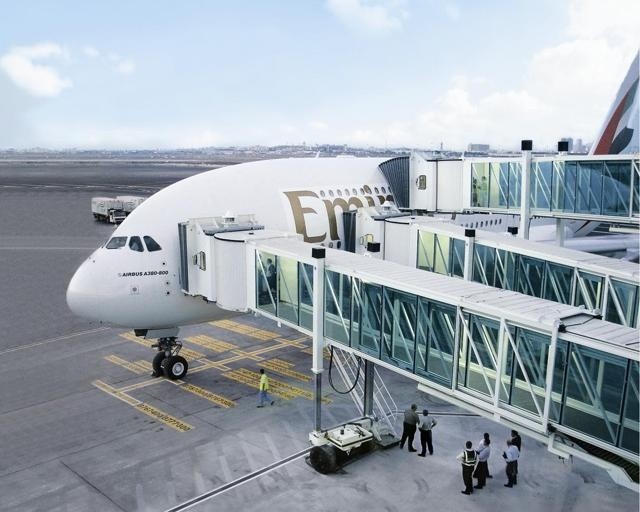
[510,430,521,474]
[399,403,420,452]
[265,258,276,300]
[503,439,520,488]
[418,409,438,457]
[257,254,266,298]
[479,433,492,478]
[456,441,477,495]
[256,369,275,408]
[473,439,490,490]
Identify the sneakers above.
[257,405,264,408]
[270,400,276,406]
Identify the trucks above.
[92,195,148,225]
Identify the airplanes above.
[66,50,640,379]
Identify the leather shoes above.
[474,484,484,489]
[461,488,474,495]
[503,482,514,488]
[487,474,494,478]
[408,447,417,452]
[417,453,426,457]
